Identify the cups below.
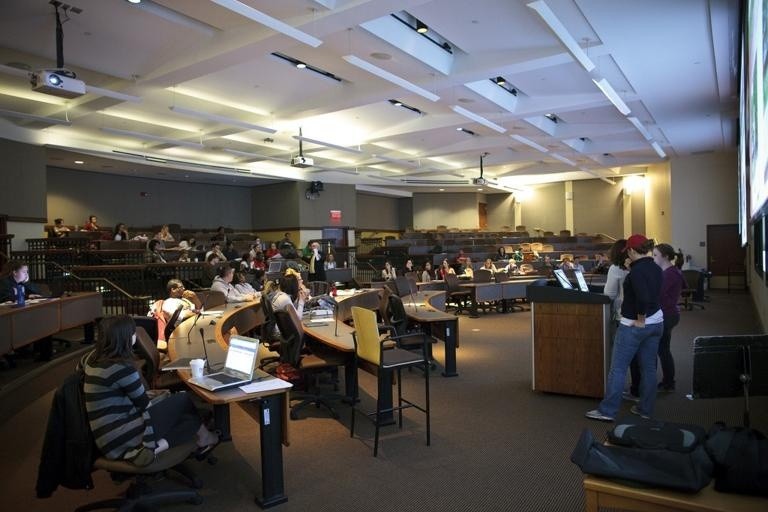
[189,359,204,379]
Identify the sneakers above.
[585,387,674,422]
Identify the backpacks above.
[570,416,727,495]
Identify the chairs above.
[402,225,615,253]
[443,253,610,277]
[676,270,705,311]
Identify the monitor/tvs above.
[552,268,573,289]
[573,268,590,292]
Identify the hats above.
[622,234,647,253]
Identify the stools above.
[729,265,747,294]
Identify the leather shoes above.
[196,429,224,461]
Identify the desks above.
[0,290,104,355]
[459,278,607,317]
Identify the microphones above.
[187,312,202,344]
[200,327,216,375]
[589,262,609,288]
[407,282,418,314]
[390,264,392,273]
[324,295,339,335]
[556,272,574,289]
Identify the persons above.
[73,312,219,457]
[652,243,688,392]
[112,223,129,240]
[382,245,607,284]
[82,215,99,232]
[583,235,665,421]
[602,237,642,404]
[74,243,103,266]
[53,218,71,237]
[129,223,337,351]
[0,258,58,356]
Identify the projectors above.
[30,70,87,99]
[472,179,488,186]
[290,157,315,167]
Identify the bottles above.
[332,284,336,296]
[17,282,25,307]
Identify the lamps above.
[295,60,306,69]
[496,77,505,85]
[416,19,428,33]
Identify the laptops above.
[36,272,64,298]
[266,261,281,273]
[188,335,260,392]
